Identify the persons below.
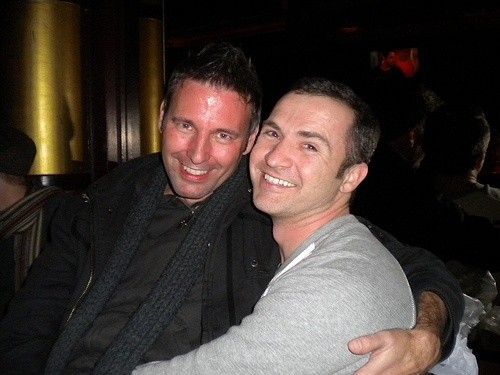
[356,80,499,274]
[0,119,67,292]
[0,41,465,375]
[127,73,416,375]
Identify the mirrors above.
[162,0,500,307]
